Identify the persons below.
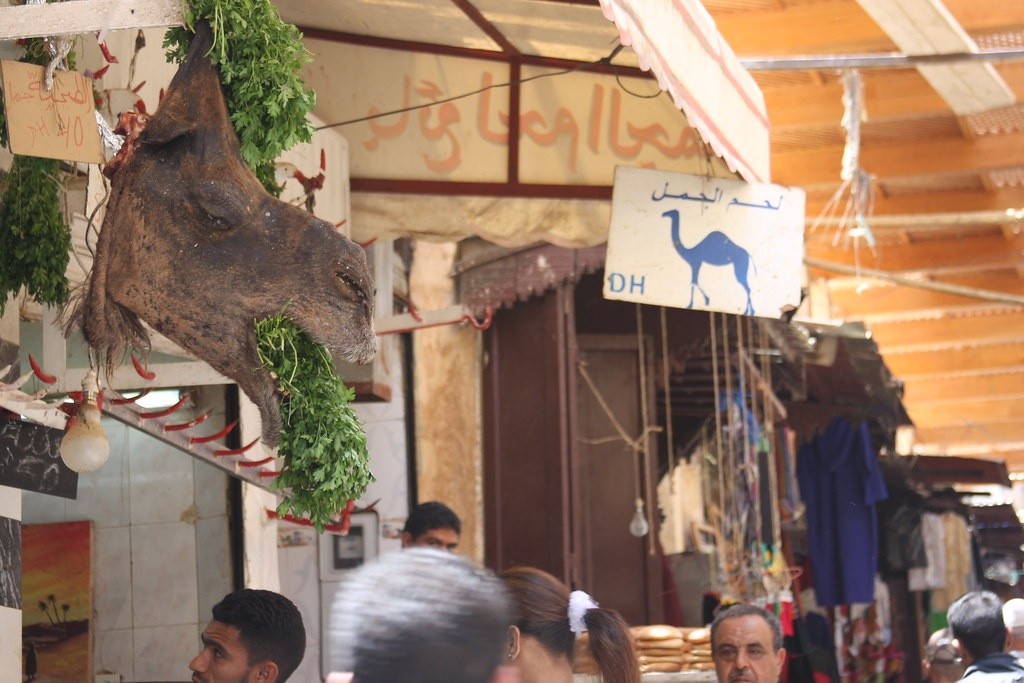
[921,589,1024,683]
[189,590,306,683]
[497,565,645,683]
[402,500,461,554]
[328,548,511,683]
[711,604,786,683]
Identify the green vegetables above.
[162,0,316,199]
[253,301,376,535]
[0,36,76,318]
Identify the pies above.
[574,624,716,673]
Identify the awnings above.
[265,0,770,249]
